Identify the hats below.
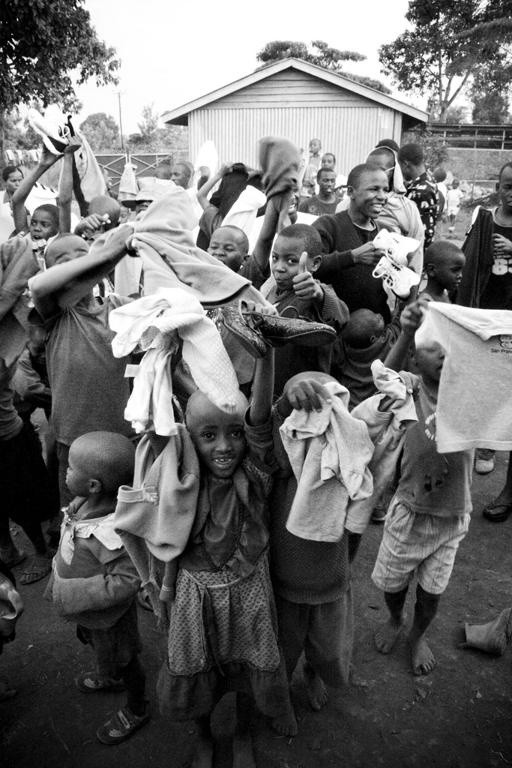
[120,189,157,209]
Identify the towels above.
[29,114,512,559]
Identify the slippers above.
[484,500,511,521]
[20,549,56,588]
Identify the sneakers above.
[371,255,422,301]
[372,228,420,267]
[96,708,151,746]
[27,102,81,158]
[372,492,394,524]
[75,669,124,695]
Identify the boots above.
[462,606,511,658]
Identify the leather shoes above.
[474,453,496,475]
[261,306,337,350]
[222,297,268,360]
